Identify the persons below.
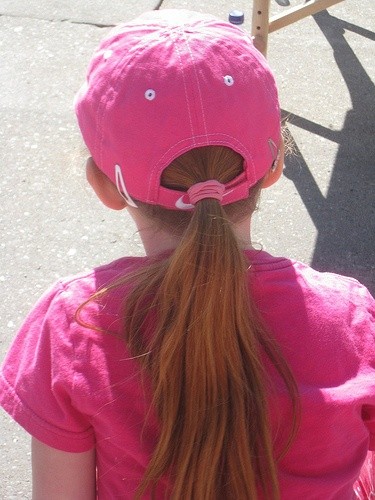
[1,12,375,500]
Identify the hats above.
[73,8,281,212]
